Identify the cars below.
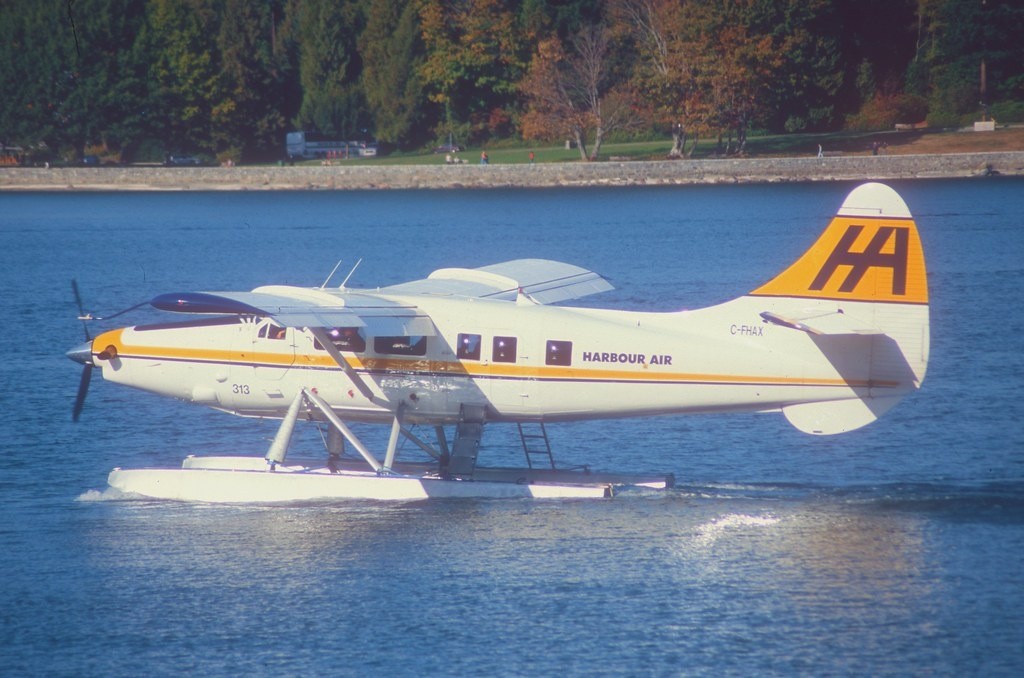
[433,143,466,156]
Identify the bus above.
[0,144,28,169]
[282,129,384,161]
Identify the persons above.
[481,150,489,164]
[288,153,295,167]
[454,156,462,164]
[43,160,51,170]
[817,143,824,158]
[446,151,453,164]
[330,327,366,353]
[528,151,535,163]
[872,140,888,156]
[275,329,288,341]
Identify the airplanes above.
[63,176,931,502]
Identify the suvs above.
[160,154,201,165]
